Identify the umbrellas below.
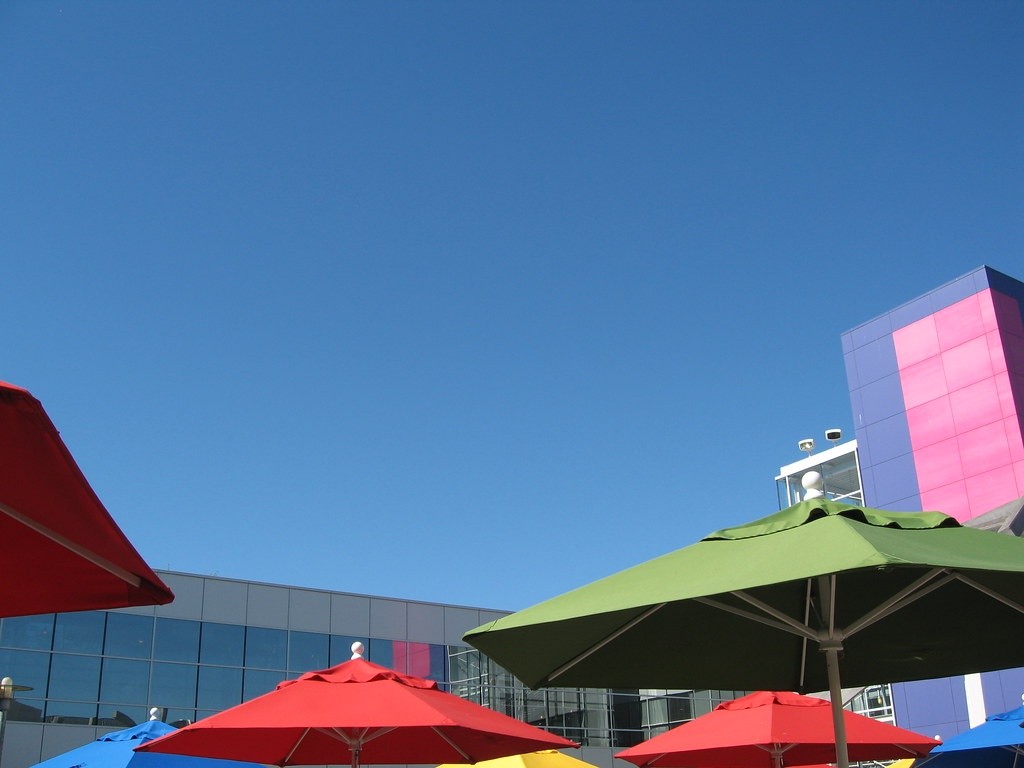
[613,692,943,768]
[910,694,1024,768]
[436,750,599,768]
[886,758,915,768]
[28,707,266,768]
[462,472,1024,768]
[132,641,581,768]
[0,382,175,617]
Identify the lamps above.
[798,438,816,458]
[825,428,842,447]
